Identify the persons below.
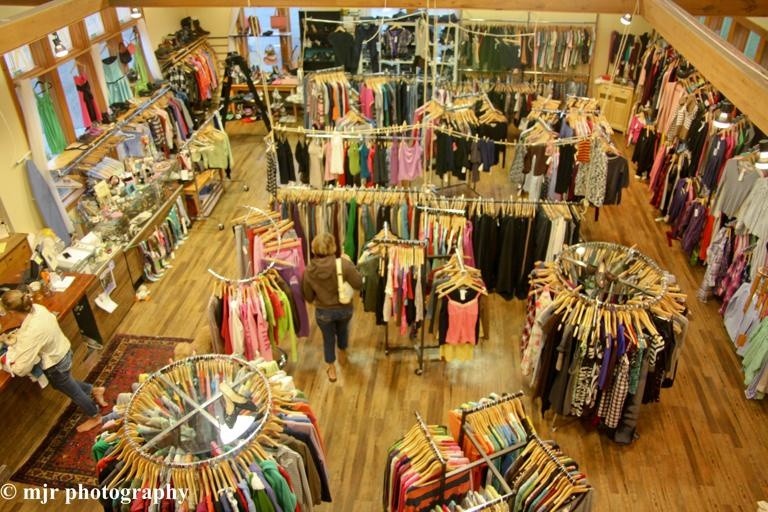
[304,233,362,380]
[0,290,108,432]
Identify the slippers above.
[327,367,337,382]
[337,353,346,366]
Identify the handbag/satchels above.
[336,257,353,305]
[271,9,288,29]
[119,42,132,64]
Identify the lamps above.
[620,13,632,26]
[129,7,143,18]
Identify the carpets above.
[8,329,195,494]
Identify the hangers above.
[52,26,766,511]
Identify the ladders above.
[219,51,270,133]
